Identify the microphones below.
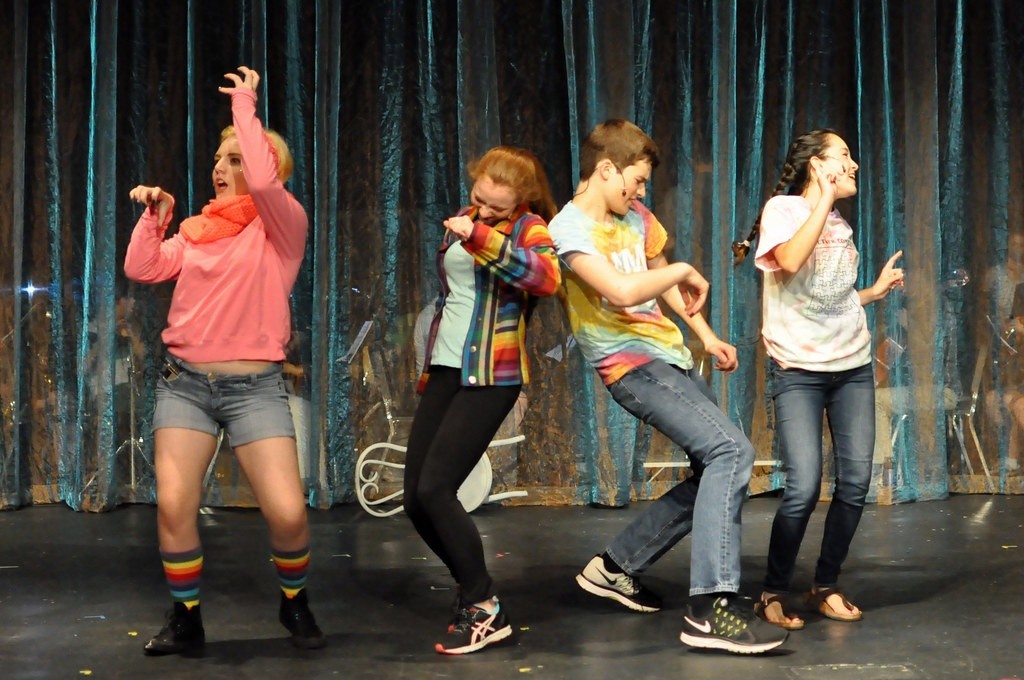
[829,156,846,173]
[613,161,626,196]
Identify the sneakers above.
[433,595,513,655]
[447,589,461,632]
[143,601,205,658]
[679,595,790,654]
[279,587,327,650]
[574,554,664,613]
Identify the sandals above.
[754,595,804,630]
[811,585,862,621]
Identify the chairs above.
[355,436,527,518]
[361,344,414,499]
[887,344,995,493]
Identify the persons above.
[85,133,963,513]
[975,231,1024,474]
[548,119,790,656]
[123,66,326,655]
[731,127,906,629]
[402,148,563,656]
